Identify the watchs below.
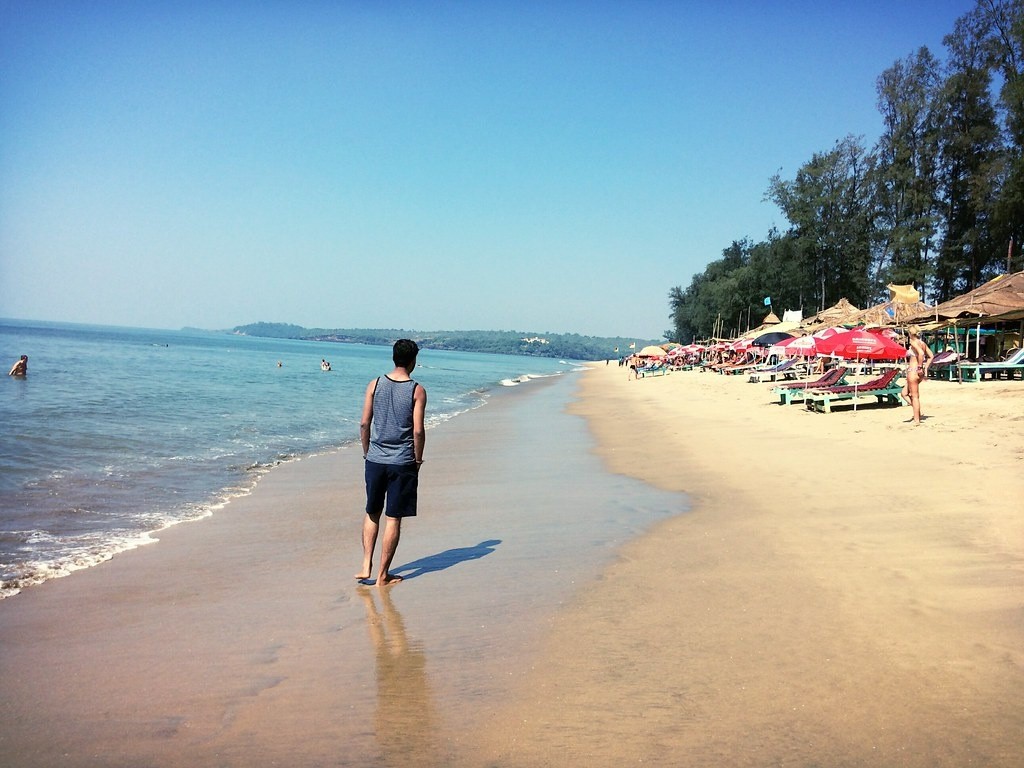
[918,367,922,370]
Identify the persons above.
[353,338,427,588]
[321,359,326,369]
[902,324,935,427]
[8,354,28,376]
[619,352,905,380]
[324,362,331,370]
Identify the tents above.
[738,270,1024,384]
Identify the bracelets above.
[416,461,423,464]
[362,454,367,459]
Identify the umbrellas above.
[814,326,908,414]
[638,326,853,361]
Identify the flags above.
[629,342,635,348]
[764,297,771,306]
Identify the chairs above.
[636,346,1024,414]
